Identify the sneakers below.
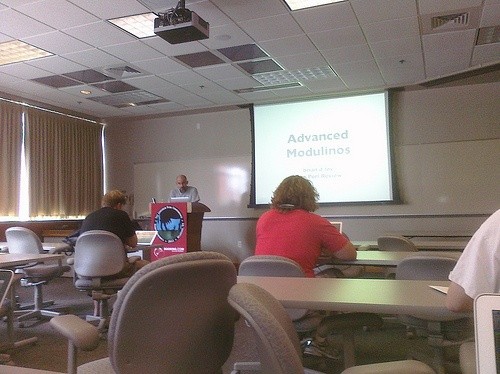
[303,339,342,360]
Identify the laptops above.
[329,221,342,234]
[170,197,190,202]
[135,230,158,245]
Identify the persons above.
[446,208,500,314]
[78,190,150,280]
[254,175,356,360]
[168,173,201,204]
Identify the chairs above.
[0,226,470,374]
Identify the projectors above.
[154,11,209,44]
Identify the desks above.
[234,275,471,321]
[316,251,463,266]
[353,237,468,251]
[1,241,70,250]
[0,254,66,354]
